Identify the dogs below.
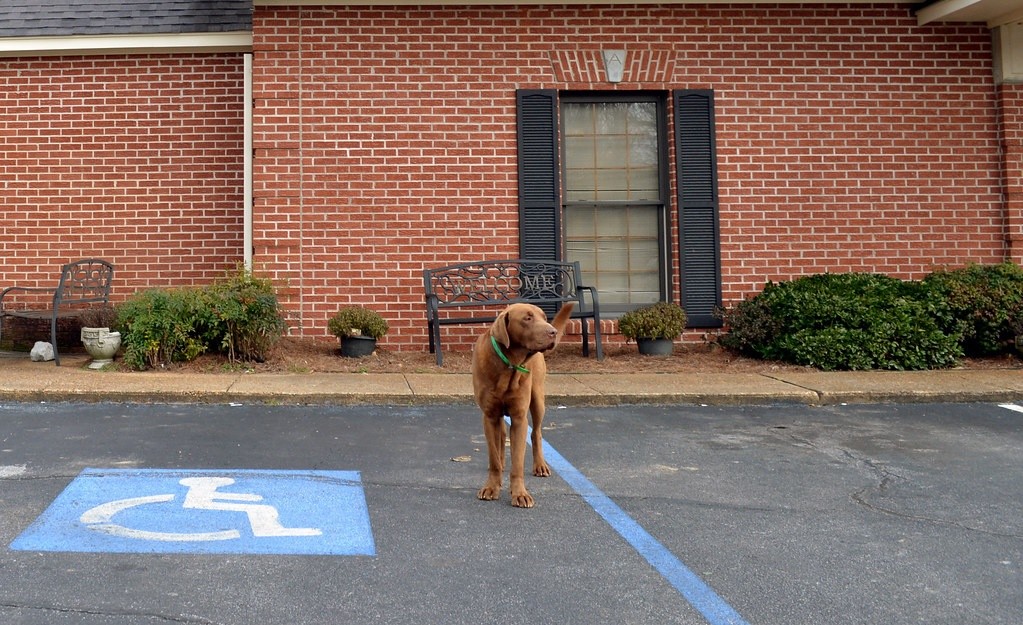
[471,301,577,508]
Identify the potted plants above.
[617,300,689,355]
[78,304,123,369]
[327,306,389,358]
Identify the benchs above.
[423,259,603,368]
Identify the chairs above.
[0,259,114,366]
